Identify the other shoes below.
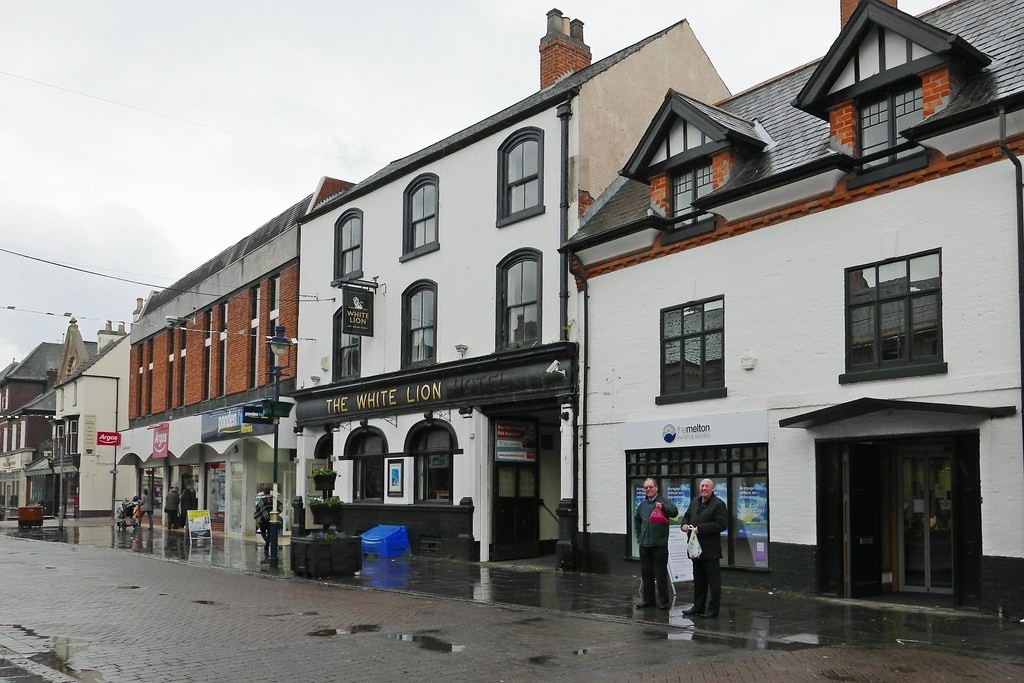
[148,527,153,530]
[265,539,270,551]
[659,602,667,608]
[682,606,705,615]
[637,602,656,608]
[700,612,718,618]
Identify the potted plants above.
[307,495,344,524]
[308,467,342,491]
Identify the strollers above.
[115,503,141,533]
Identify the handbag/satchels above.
[686,524,702,558]
[650,501,669,524]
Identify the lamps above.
[424,410,434,419]
[359,419,368,427]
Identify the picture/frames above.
[387,459,404,497]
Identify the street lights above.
[263,325,292,568]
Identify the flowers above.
[306,529,351,541]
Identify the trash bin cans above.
[18,506,43,526]
[361,524,410,558]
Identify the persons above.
[634,478,678,610]
[164,487,196,530]
[253,489,284,549]
[680,478,729,618]
[137,488,153,529]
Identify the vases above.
[289,536,363,579]
